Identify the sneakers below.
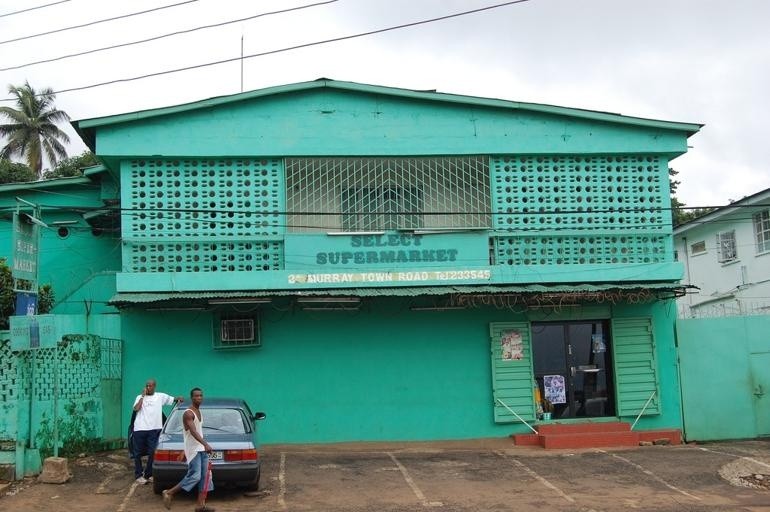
[162,490,173,510]
[195,506,215,512]
[136,475,153,485]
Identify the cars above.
[152,397,266,494]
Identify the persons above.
[161,387,216,511]
[129,380,186,484]
